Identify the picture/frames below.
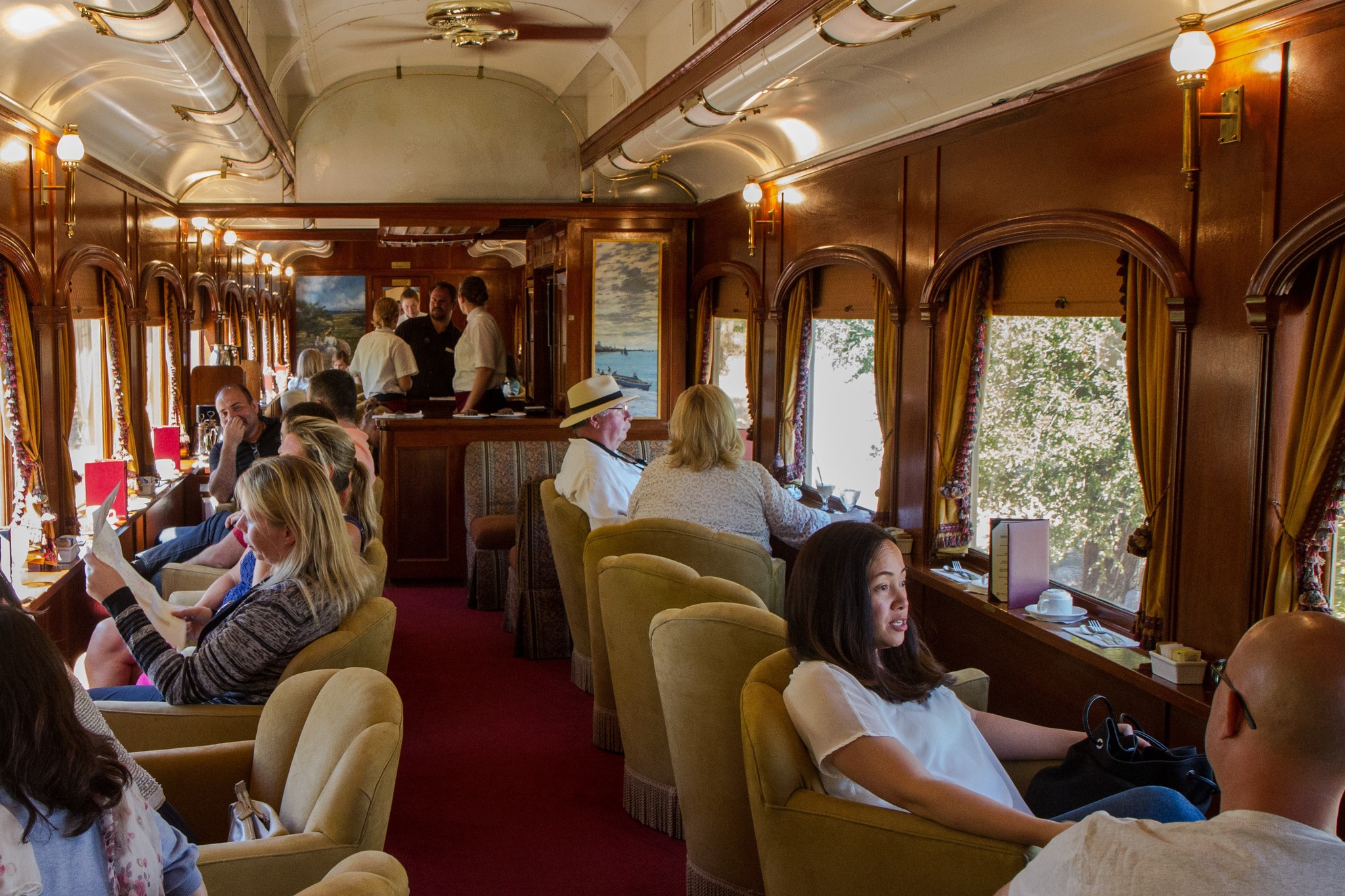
[589,237,663,422]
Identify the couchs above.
[73,474,1120,896]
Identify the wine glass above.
[838,485,861,511]
[816,477,836,511]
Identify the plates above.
[452,414,489,419]
[971,579,988,589]
[1025,604,1088,621]
[491,412,527,417]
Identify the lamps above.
[742,172,776,257]
[182,216,294,296]
[1168,14,1244,187]
[39,121,86,240]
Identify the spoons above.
[1080,624,1116,645]
[943,565,968,580]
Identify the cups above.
[1037,589,1073,615]
[86,505,116,524]
[155,459,175,475]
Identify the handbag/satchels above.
[1022,694,1221,820]
[227,780,290,843]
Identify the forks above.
[1088,620,1127,645]
[952,561,978,580]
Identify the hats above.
[559,375,639,428]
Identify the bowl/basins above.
[1149,650,1208,684]
[58,543,78,563]
[895,539,913,553]
[140,481,156,495]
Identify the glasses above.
[251,441,260,461]
[1206,657,1256,730]
[606,405,628,412]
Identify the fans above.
[343,0,612,50]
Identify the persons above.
[393,283,465,398]
[451,275,509,416]
[83,345,382,709]
[992,611,1345,896]
[553,374,649,529]
[782,520,1209,850]
[627,384,869,556]
[347,296,419,411]
[1,599,209,896]
[393,289,430,334]
